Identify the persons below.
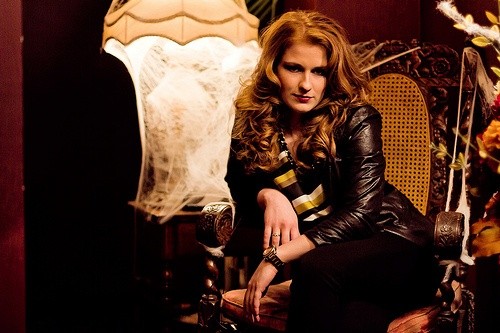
[222,11,433,333]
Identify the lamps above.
[103,0,261,212]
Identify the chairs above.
[198,40,475,332]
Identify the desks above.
[126,201,205,333]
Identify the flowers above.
[429,0,500,226]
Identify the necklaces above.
[276,120,324,173]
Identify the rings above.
[272,232,280,237]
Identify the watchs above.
[263,245,285,271]
